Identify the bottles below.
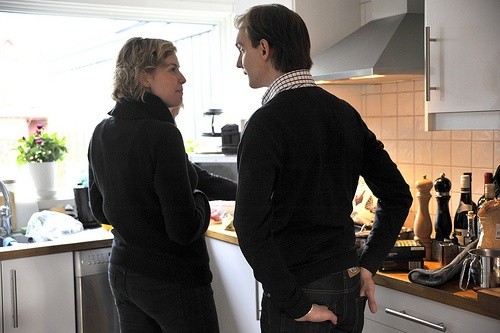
[413,171,496,260]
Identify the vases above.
[28,161,56,196]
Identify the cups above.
[73,187,100,230]
[459,248,500,291]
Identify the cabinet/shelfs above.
[205,236,500,333]
[424,0,500,132]
[0,251,75,333]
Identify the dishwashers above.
[74,247,120,333]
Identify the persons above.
[168,97,237,202]
[232,4,413,333]
[88,37,220,333]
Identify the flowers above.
[9,123,69,166]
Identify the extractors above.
[309,0,424,85]
